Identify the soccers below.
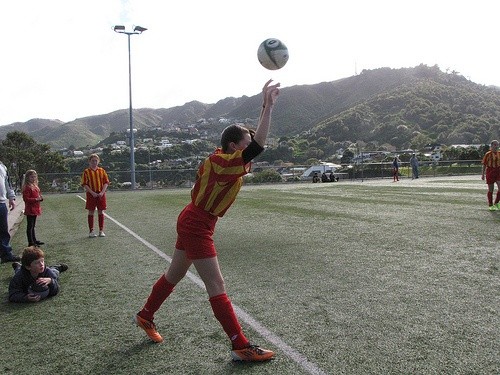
[257,38,289,71]
[27,281,49,299]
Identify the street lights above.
[114,24,148,190]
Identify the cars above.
[73,151,84,159]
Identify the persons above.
[313,172,336,183]
[134,79,280,363]
[81,154,109,238]
[22,170,45,247]
[481,140,500,210]
[0,161,21,263]
[410,154,419,178]
[393,157,399,182]
[10,246,68,303]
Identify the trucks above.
[282,168,307,182]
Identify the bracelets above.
[263,105,265,107]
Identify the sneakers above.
[99,231,106,236]
[231,344,275,362]
[89,233,97,237]
[488,202,500,211]
[136,311,163,343]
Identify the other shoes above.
[49,264,68,273]
[12,262,22,270]
[1,255,23,262]
[28,240,44,246]
